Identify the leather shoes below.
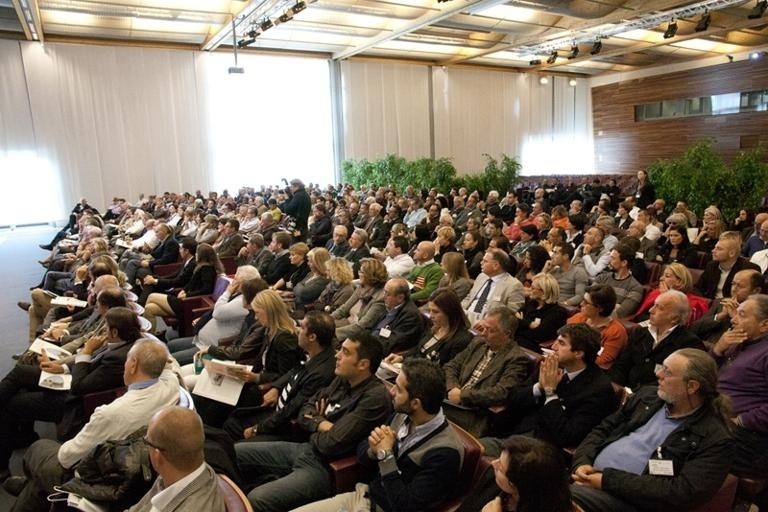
[39,245,53,251]
[38,260,54,268]
[0,470,27,496]
[18,302,31,311]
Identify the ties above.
[474,279,493,313]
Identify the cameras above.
[279,178,290,194]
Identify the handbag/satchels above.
[48,486,107,512]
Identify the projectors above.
[228,67,244,73]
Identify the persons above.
[0,168,768,512]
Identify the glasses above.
[143,433,165,452]
